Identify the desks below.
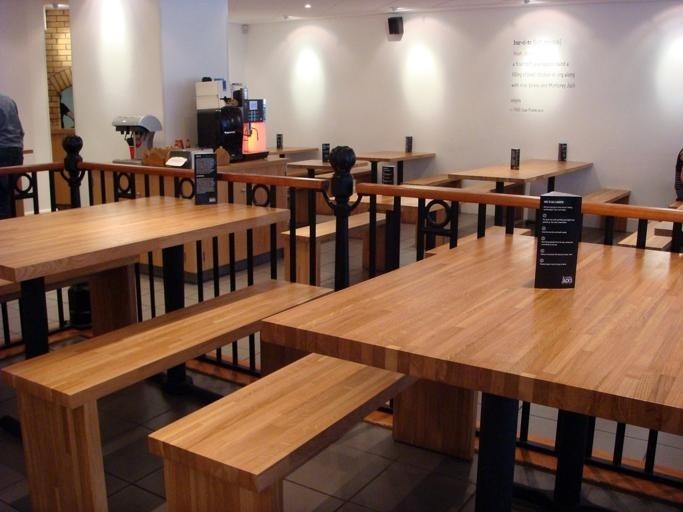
[259,234,683,512]
[1,195,290,404]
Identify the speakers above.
[388,17,403,35]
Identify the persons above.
[60,94,74,129]
[671,149,683,253]
[0,94,24,220]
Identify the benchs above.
[149,352,479,512]
[110,147,683,283]
[0,280,334,512]
